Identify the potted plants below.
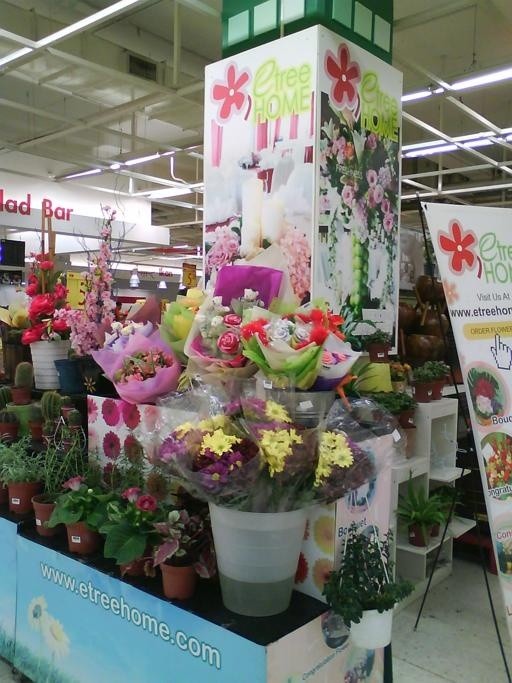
[1,361,102,539]
[427,486,461,522]
[47,475,207,604]
[393,489,439,546]
[322,490,413,650]
[426,499,442,537]
[362,326,391,366]
[20,201,137,396]
[407,365,432,402]
[423,361,449,400]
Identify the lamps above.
[393,0,512,203]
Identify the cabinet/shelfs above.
[10,530,385,680]
[391,391,475,615]
[0,501,16,672]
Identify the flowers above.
[165,400,363,510]
[91,266,366,396]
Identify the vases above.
[397,267,453,365]
[207,492,310,620]
